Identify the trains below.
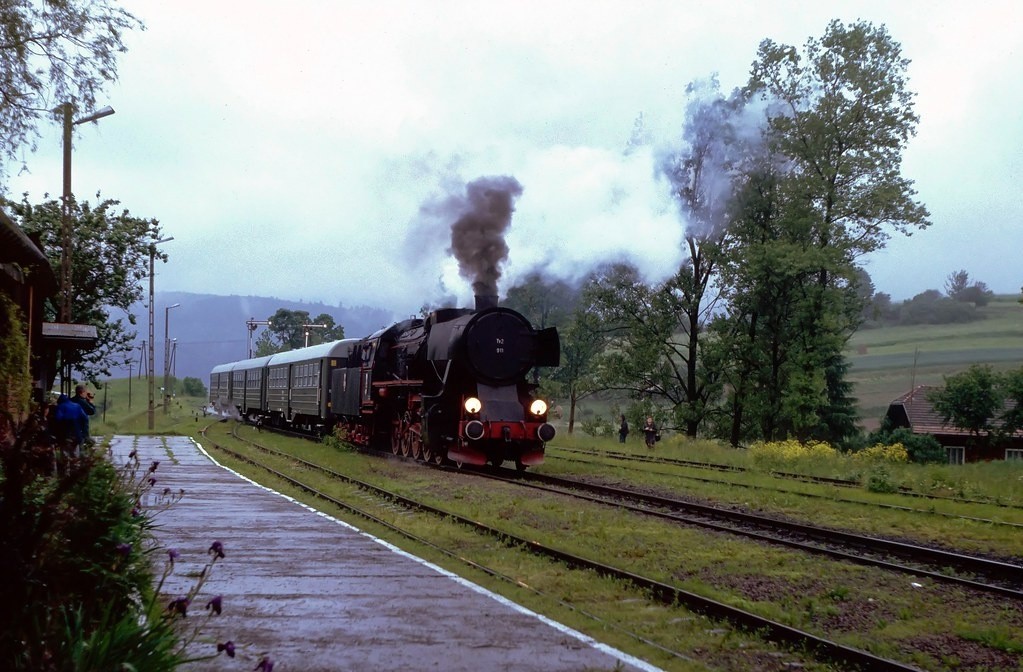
[209,295,560,480]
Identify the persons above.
[28,380,97,459]
[618,415,628,443]
[642,416,657,449]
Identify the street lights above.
[147,237,177,429]
[301,324,327,348]
[163,303,180,413]
[246,318,272,358]
[62,102,115,395]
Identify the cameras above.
[87,393,95,398]
